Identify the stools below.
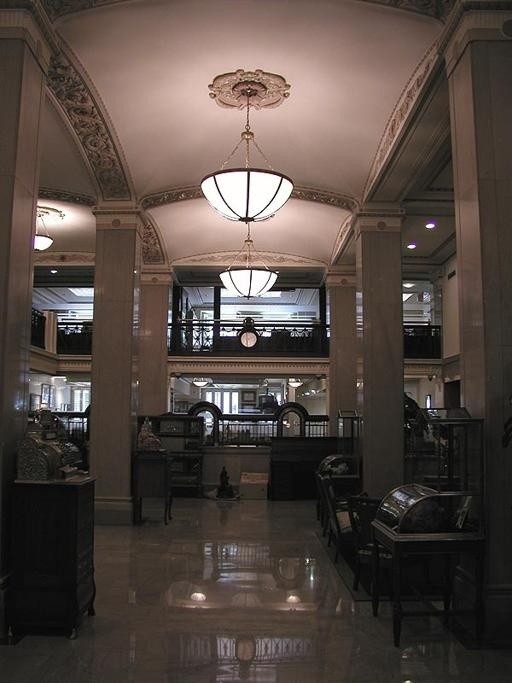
[134,449,173,525]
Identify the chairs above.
[312,473,333,547]
[322,474,362,563]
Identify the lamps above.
[33,212,55,252]
[201,68,295,299]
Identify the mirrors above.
[239,317,260,351]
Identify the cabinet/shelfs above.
[370,483,486,648]
[141,414,203,498]
[318,455,359,493]
[7,475,98,645]
